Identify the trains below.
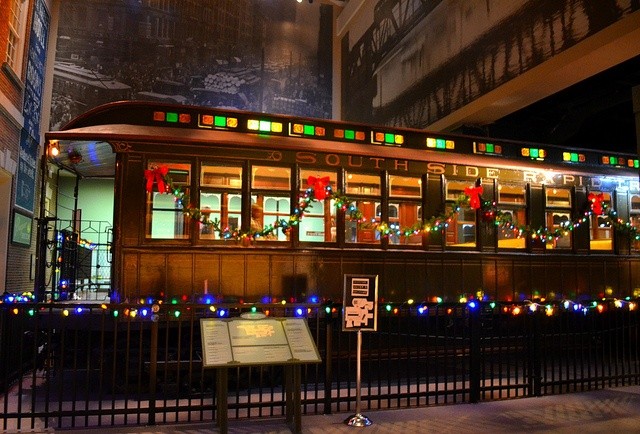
[34,101,640,400]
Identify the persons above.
[251,203,272,239]
[199,205,214,235]
